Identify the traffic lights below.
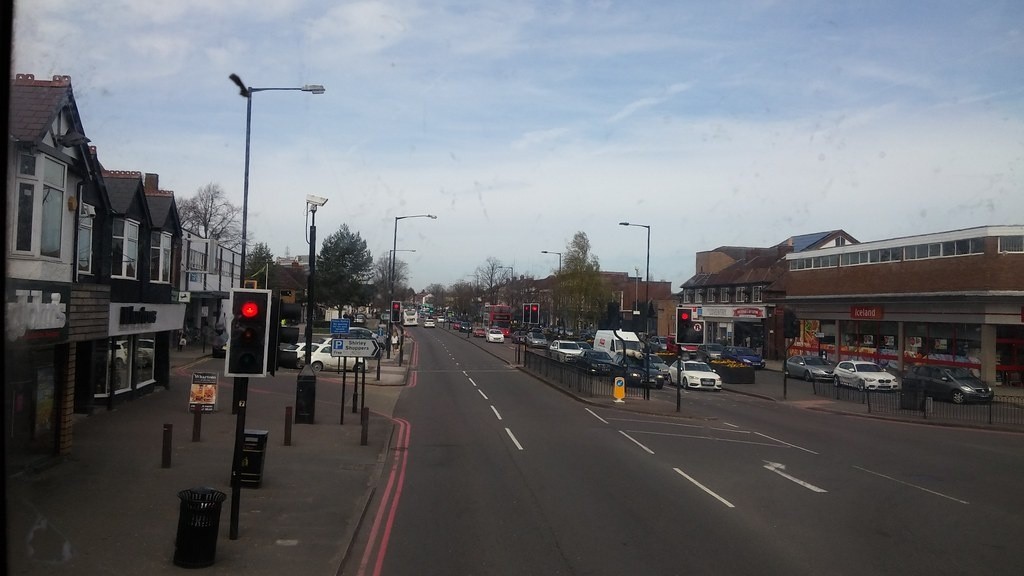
[675,306,705,346]
[529,302,540,325]
[523,303,531,323]
[223,287,274,379]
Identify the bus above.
[401,308,419,326]
[482,304,513,338]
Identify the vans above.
[667,335,698,359]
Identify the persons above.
[392,332,398,354]
[281,317,287,326]
[178,329,187,351]
[343,312,354,322]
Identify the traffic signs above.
[330,338,380,357]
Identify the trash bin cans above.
[174,487,227,567]
[240,429,267,488]
[899,377,927,411]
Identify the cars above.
[642,353,669,380]
[473,327,486,338]
[720,346,766,370]
[458,322,473,333]
[486,328,506,344]
[137,338,154,368]
[354,315,367,324]
[424,319,435,328]
[667,360,724,392]
[282,327,387,373]
[420,308,463,329]
[610,352,665,391]
[832,359,900,393]
[549,340,583,362]
[648,336,668,353]
[576,349,613,374]
[511,331,527,344]
[577,341,593,351]
[697,343,725,364]
[540,323,597,340]
[524,332,548,349]
[108,339,128,369]
[781,355,835,383]
[901,363,994,404]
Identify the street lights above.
[386,248,416,308]
[229,72,326,414]
[497,264,514,307]
[390,214,437,303]
[619,221,650,334]
[541,250,562,307]
[390,300,403,324]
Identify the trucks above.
[593,329,643,358]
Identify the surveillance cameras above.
[306,194,329,206]
